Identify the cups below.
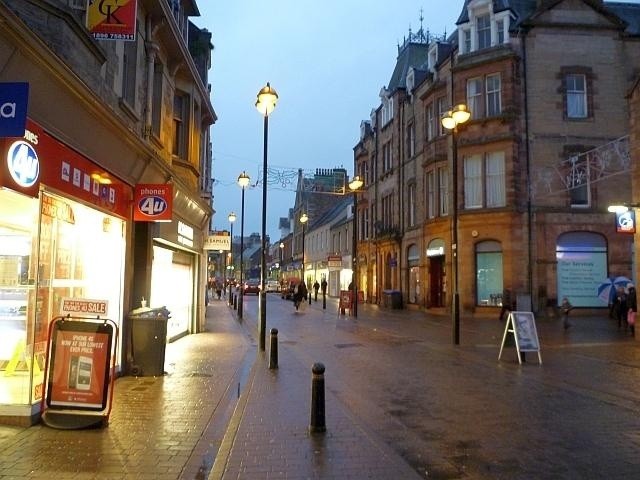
[141,300,147,308]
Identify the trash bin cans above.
[124,306,172,376]
[382,291,402,309]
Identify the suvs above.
[281,276,308,302]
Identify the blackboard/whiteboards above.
[511,312,541,352]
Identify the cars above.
[225,276,284,296]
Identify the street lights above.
[227,211,238,308]
[279,241,285,286]
[235,170,251,320]
[300,214,309,283]
[439,101,472,347]
[253,81,279,355]
[348,174,365,322]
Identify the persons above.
[321,278,327,293]
[614,286,629,333]
[626,283,637,338]
[293,279,302,312]
[216,276,222,299]
[560,297,577,331]
[313,280,320,293]
[499,285,514,322]
[300,282,310,303]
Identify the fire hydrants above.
[558,297,576,330]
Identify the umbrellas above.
[595,275,634,305]
